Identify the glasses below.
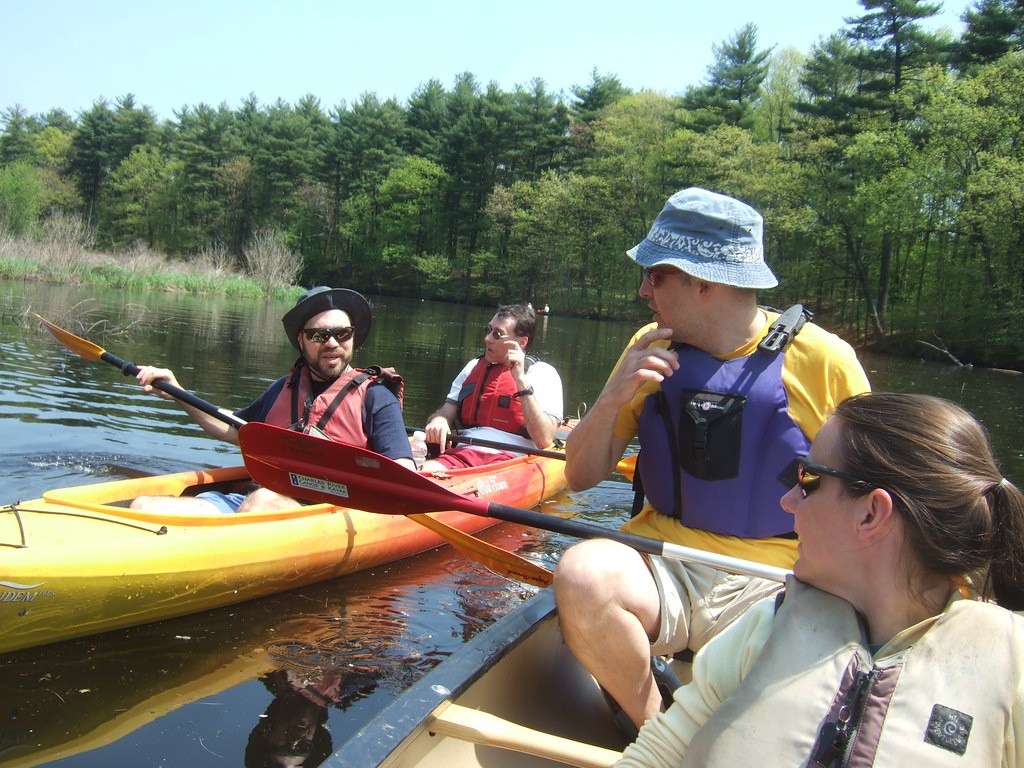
[300,326,355,343]
[484,325,513,340]
[643,267,683,287]
[793,456,876,499]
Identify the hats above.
[282,286,373,350]
[625,187,779,289]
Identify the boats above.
[0,403,589,654]
[318,586,695,768]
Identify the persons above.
[418,304,563,472]
[553,186,872,732]
[131,285,416,516]
[542,304,549,312]
[608,391,1024,768]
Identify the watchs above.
[518,385,534,396]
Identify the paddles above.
[402,420,642,481]
[31,312,556,590]
[234,417,799,584]
